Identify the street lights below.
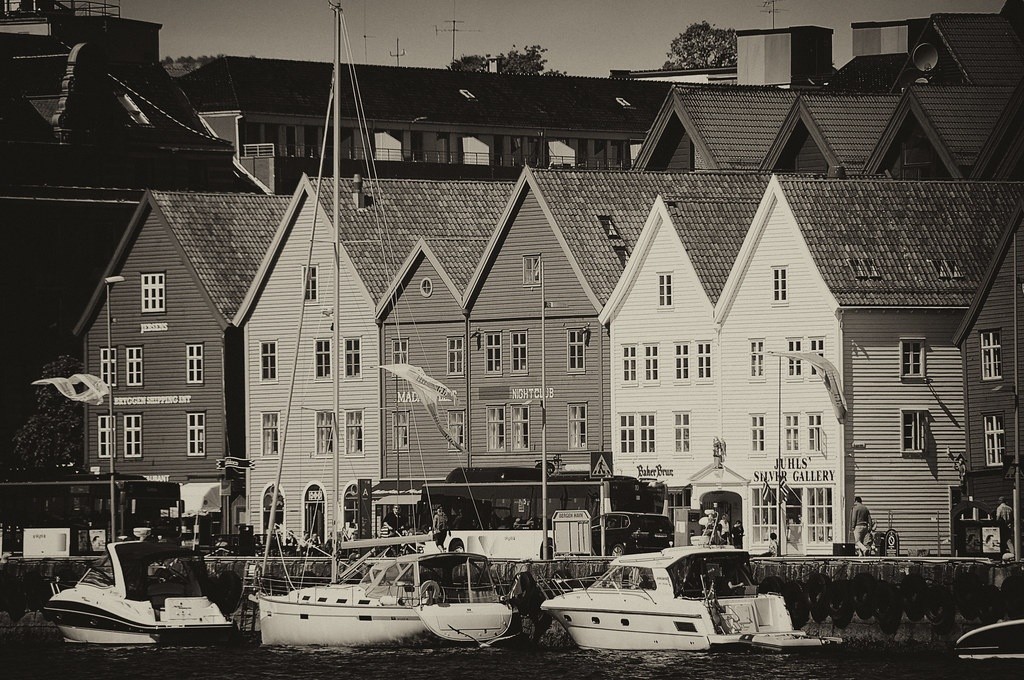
[103,275,126,541]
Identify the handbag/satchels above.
[432,515,441,536]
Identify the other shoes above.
[864,549,869,556]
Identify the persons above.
[716,513,730,544]
[382,505,406,557]
[759,533,777,557]
[434,506,451,552]
[996,497,1013,554]
[732,521,743,548]
[703,514,717,546]
[277,524,357,557]
[852,497,872,556]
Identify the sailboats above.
[258,0,521,650]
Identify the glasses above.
[393,507,398,509]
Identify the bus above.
[418,466,669,560]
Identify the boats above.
[43,540,239,646]
[539,544,845,650]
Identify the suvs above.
[591,511,674,556]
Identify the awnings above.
[374,494,422,506]
[178,483,221,514]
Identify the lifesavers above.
[420,581,443,604]
[928,585,955,636]
[784,582,808,628]
[877,581,903,634]
[833,579,854,628]
[510,572,536,607]
[808,573,832,620]
[855,574,875,620]
[0,568,79,614]
[1003,576,1024,620]
[550,571,575,596]
[759,577,783,594]
[956,574,979,620]
[980,585,1002,626]
[905,574,927,621]
